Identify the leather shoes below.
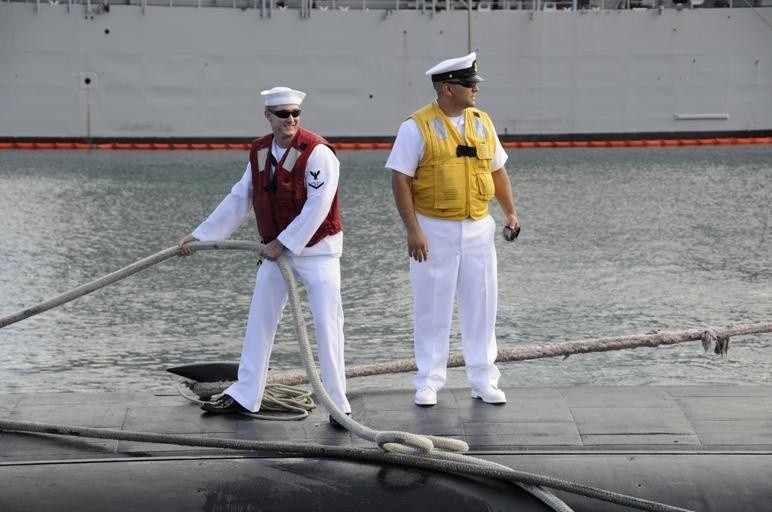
[328,414,354,430]
[470,382,507,404]
[201,394,259,415]
[415,384,437,405]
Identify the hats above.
[425,52,484,83]
[260,87,306,106]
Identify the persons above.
[174,85,355,434]
[382,50,522,407]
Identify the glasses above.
[270,108,302,117]
[444,81,479,87]
